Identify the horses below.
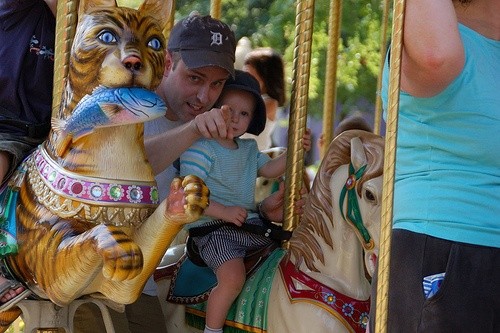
[153,129,386,333]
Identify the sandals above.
[0,277,31,314]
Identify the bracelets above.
[256,199,269,221]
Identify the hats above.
[225,69,266,136]
[167,15,236,81]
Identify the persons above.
[381,0,500,333]
[175,72,287,330]
[238,48,286,153]
[138,13,234,208]
[0,0,56,191]
